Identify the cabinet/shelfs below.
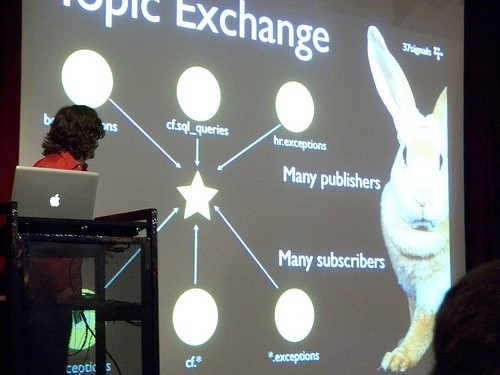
[0,201,159,375]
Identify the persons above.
[8,105,107,375]
[430,258,500,375]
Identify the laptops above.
[11,165,99,220]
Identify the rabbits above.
[366,21,451,372]
